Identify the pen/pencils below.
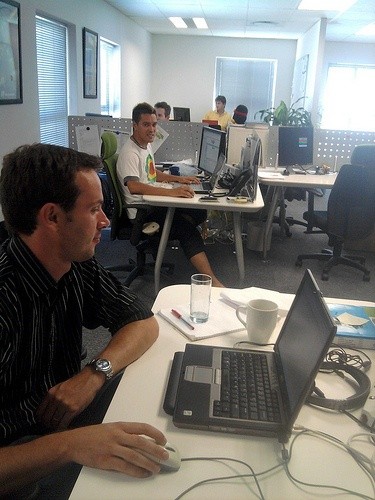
[196,150,198,163]
[170,308,195,330]
[284,163,290,171]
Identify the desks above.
[142,171,265,299]
[258,167,340,257]
[68,285,375,500]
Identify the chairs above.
[296,164,375,282]
[352,145,375,168]
[101,131,174,287]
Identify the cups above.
[190,274,212,324]
[236,299,279,345]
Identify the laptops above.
[172,153,226,193]
[162,268,337,434]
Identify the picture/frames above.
[83,27,99,98]
[0,0,23,105]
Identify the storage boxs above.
[326,303,375,349]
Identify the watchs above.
[85,357,113,381]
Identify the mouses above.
[122,436,181,471]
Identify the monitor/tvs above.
[173,107,190,121]
[279,126,313,174]
[198,126,226,175]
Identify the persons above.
[154,102,171,120]
[116,103,227,289]
[202,96,232,129]
[0,144,167,500]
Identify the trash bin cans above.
[246,222,273,252]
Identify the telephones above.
[228,168,252,197]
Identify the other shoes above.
[141,221,159,236]
